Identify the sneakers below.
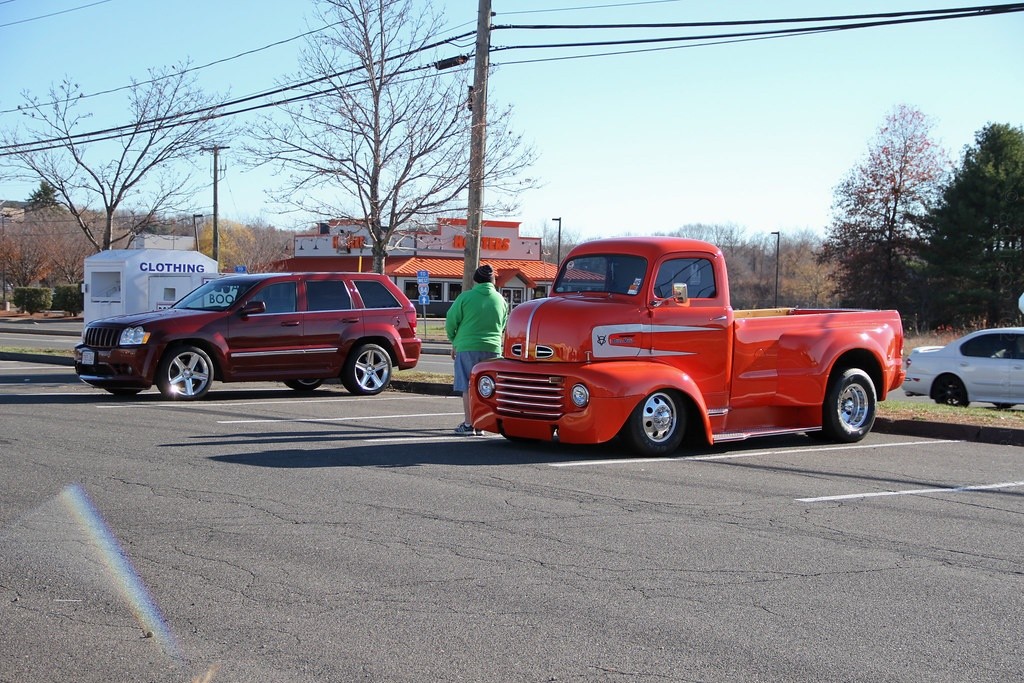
[475,429,485,435]
[453,421,476,435]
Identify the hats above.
[472,264,496,285]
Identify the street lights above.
[192,214,204,251]
[771,232,781,309]
[552,217,562,272]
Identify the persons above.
[446,265,508,435]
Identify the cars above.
[901,327,1024,408]
[72,273,423,402]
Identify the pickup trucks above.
[467,236,902,458]
